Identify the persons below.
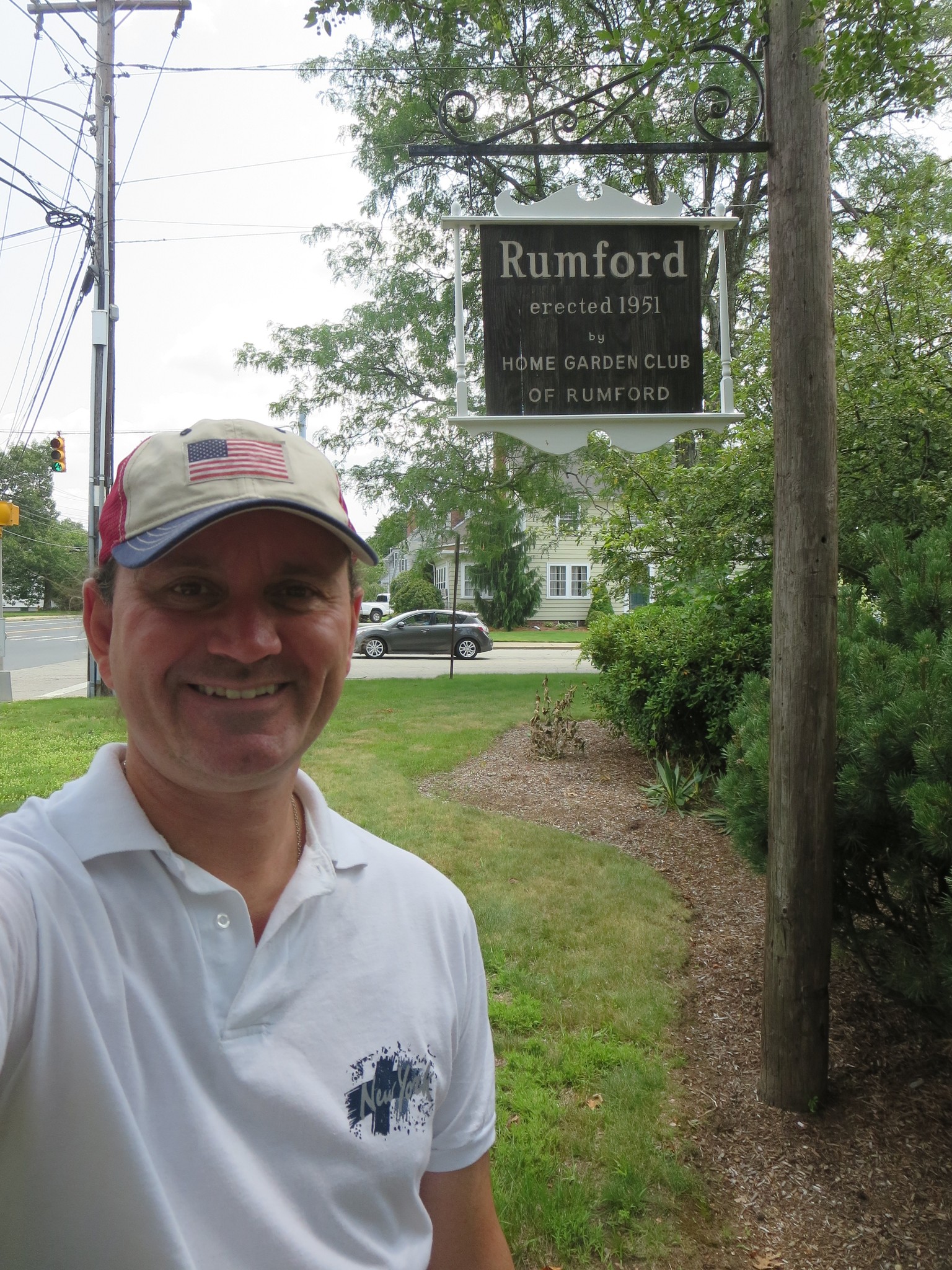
[0,415,528,1269]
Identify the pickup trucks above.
[359,593,397,623]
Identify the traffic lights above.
[49,436,67,473]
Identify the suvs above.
[352,609,495,660]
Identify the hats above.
[97,418,380,569]
